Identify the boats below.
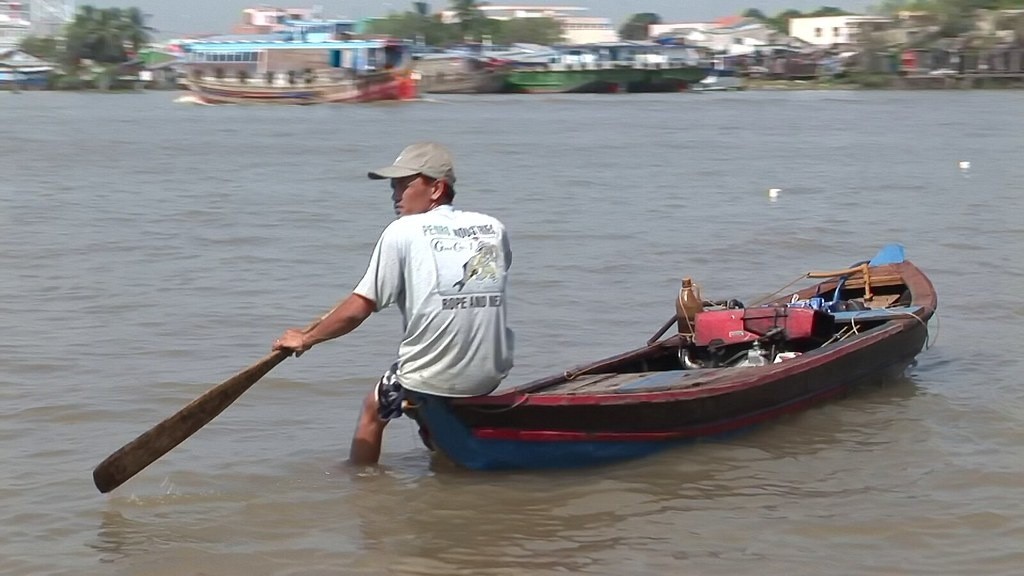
[182,65,425,104]
[401,243,940,475]
[405,51,716,95]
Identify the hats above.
[368,143,455,188]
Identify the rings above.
[276,338,279,342]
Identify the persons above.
[273,144,515,464]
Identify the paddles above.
[92,296,353,494]
